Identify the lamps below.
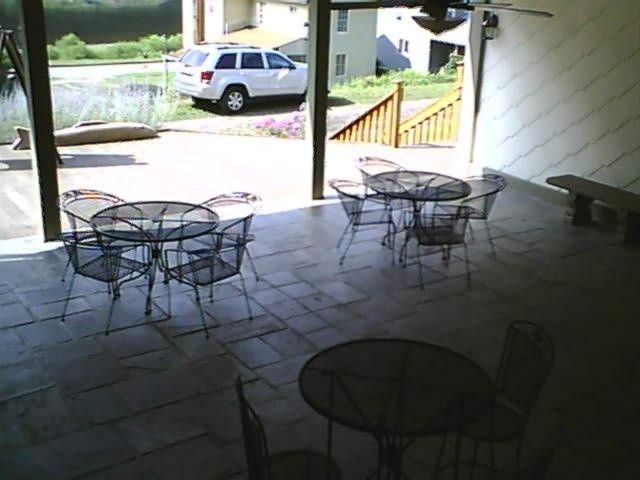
[410,5,467,36]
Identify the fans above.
[328,0,555,36]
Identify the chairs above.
[55,230,151,336]
[236,376,343,480]
[431,171,509,267]
[164,214,254,338]
[356,155,423,246]
[329,179,398,265]
[176,191,259,303]
[55,188,147,294]
[434,318,551,480]
[400,207,487,290]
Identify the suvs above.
[171,42,331,115]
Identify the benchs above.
[546,174,639,232]
